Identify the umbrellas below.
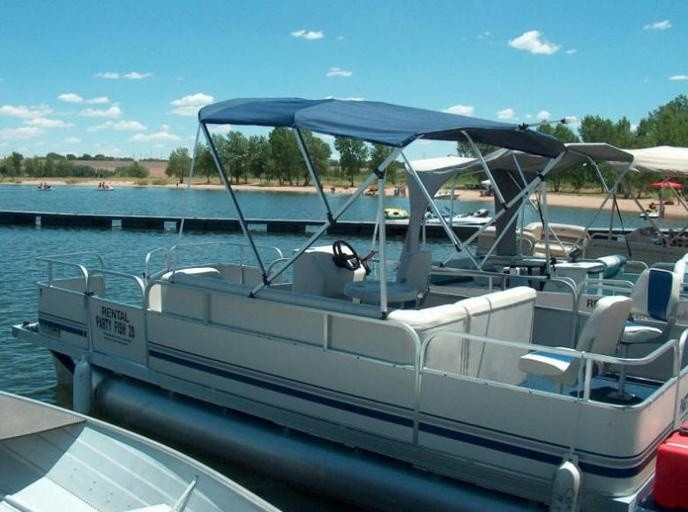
[649,181,680,189]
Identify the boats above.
[0,392,282,512]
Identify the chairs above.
[343,252,688,386]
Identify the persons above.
[423,204,432,219]
